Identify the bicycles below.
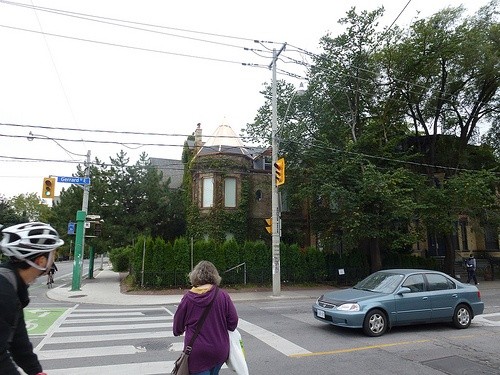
[46,269,57,289]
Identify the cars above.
[311,268,484,338]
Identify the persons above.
[172,261,238,375]
[47,263,58,285]
[0,221,64,375]
[466,254,480,285]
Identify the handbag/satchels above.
[171,353,189,375]
[227,327,249,375]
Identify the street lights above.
[26,131,91,289]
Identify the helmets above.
[0,222,64,262]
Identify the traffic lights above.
[94,222,101,236]
[274,158,285,186]
[42,178,55,198]
[264,217,272,236]
